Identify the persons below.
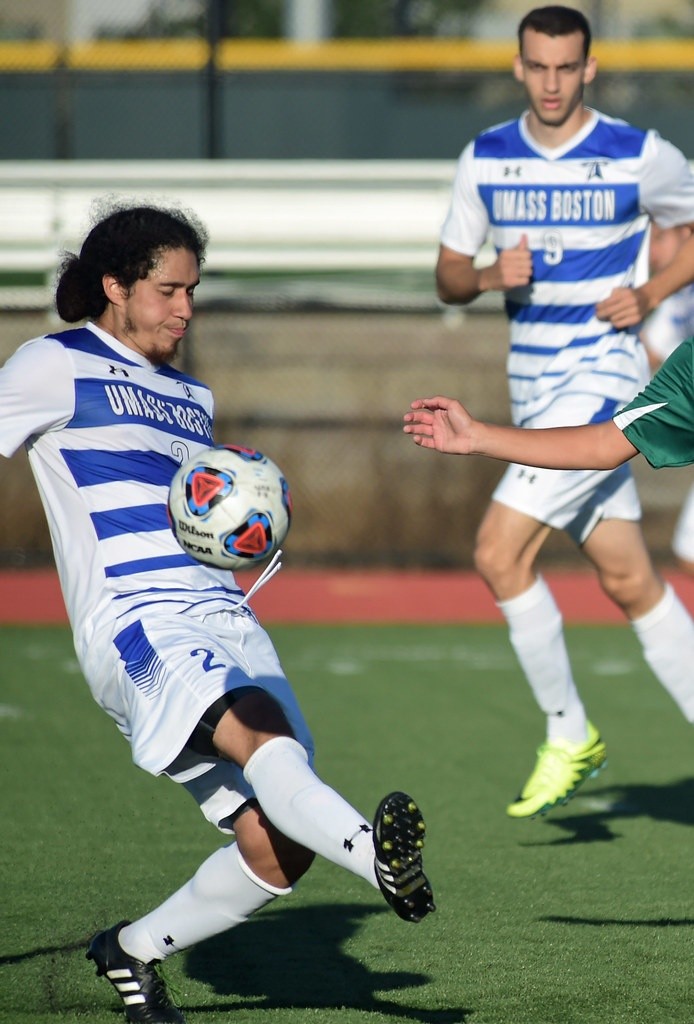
[0,205,436,1024]
[402,6,694,820]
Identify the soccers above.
[166,442,295,572]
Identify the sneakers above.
[506,721,608,820]
[372,791,436,923]
[86,920,186,1024]
